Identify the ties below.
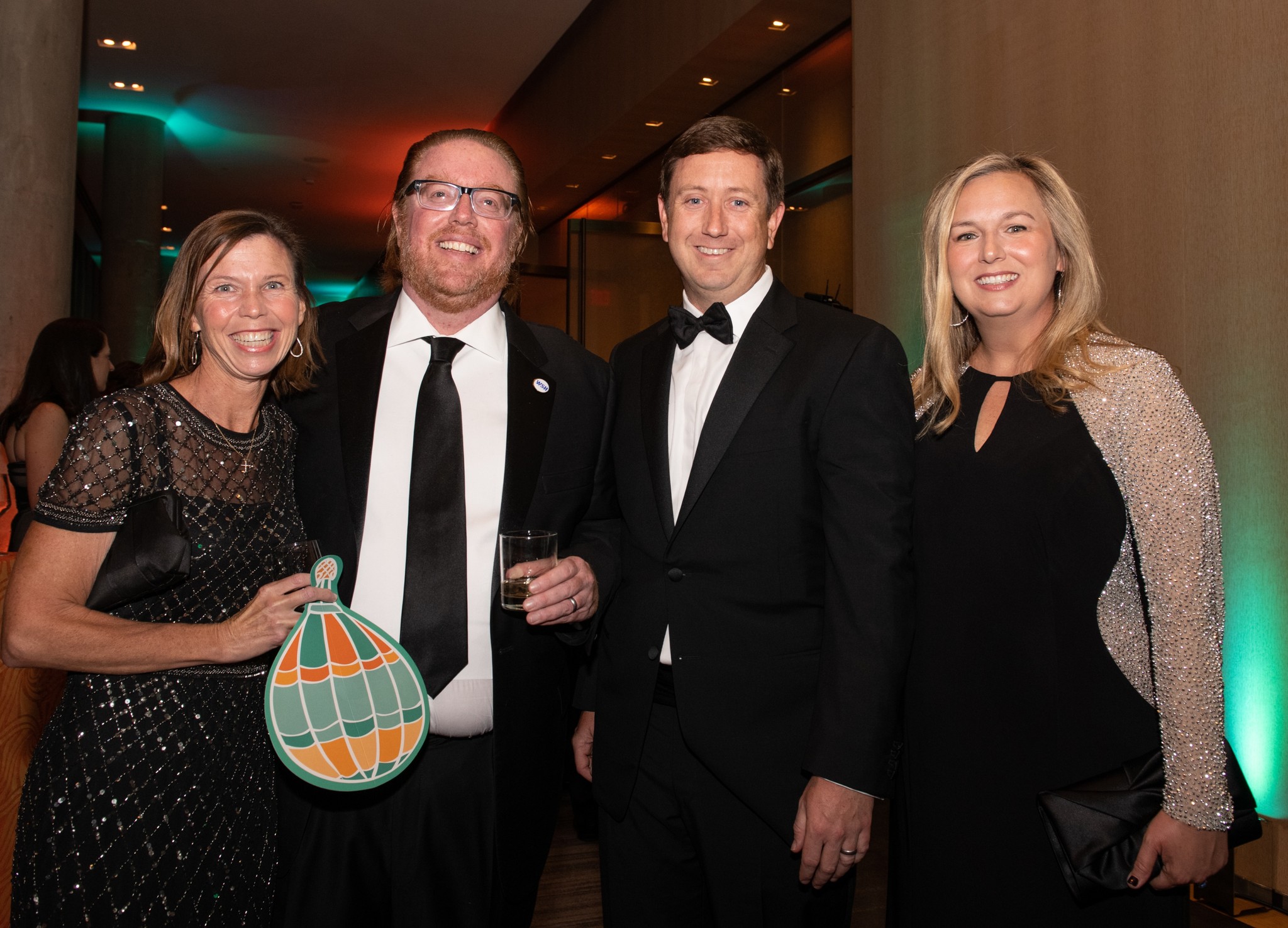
[400,336,469,701]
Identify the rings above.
[569,597,578,613]
[840,848,857,855]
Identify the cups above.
[499,530,558,610]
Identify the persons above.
[275,129,611,928]
[0,210,325,928]
[569,117,918,928]
[887,154,1263,928]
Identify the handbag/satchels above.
[83,489,192,610]
[1040,735,1263,899]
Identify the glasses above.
[397,179,523,219]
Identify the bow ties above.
[668,302,734,351]
